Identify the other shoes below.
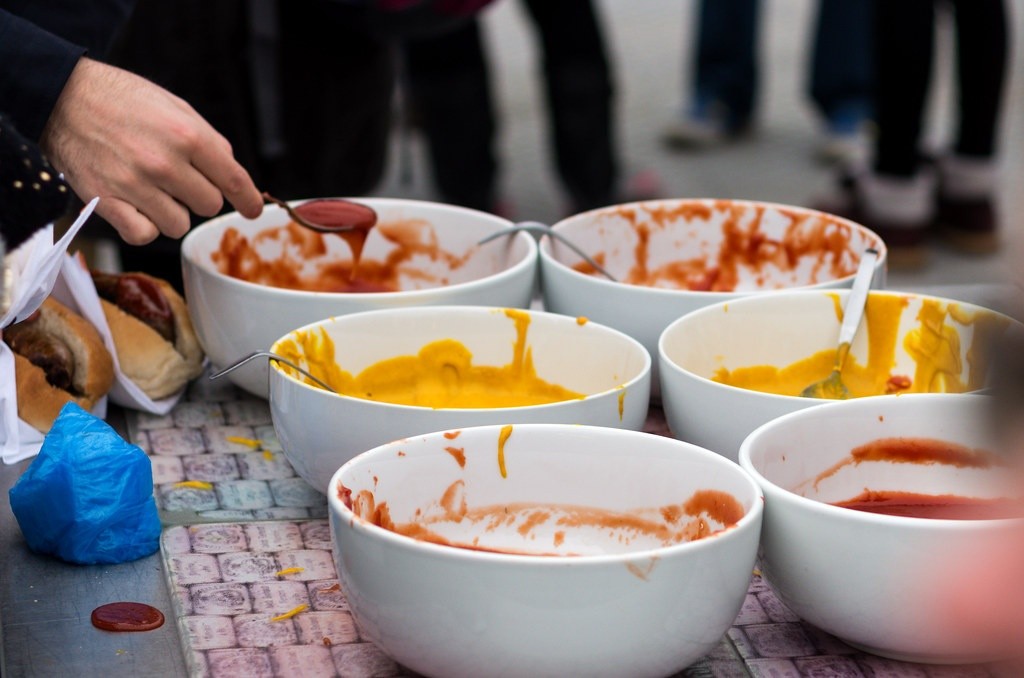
[857,170,936,271]
[662,99,758,144]
[801,144,868,213]
[936,157,1001,256]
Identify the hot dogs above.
[92,272,204,401]
[0,293,115,435]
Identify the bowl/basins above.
[538,198,888,406]
[657,288,1024,467]
[269,303,651,495]
[739,392,1024,664]
[328,424,764,678]
[181,197,537,403]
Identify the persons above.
[0,0,1024,245]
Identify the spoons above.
[259,193,375,231]
[800,249,878,398]
